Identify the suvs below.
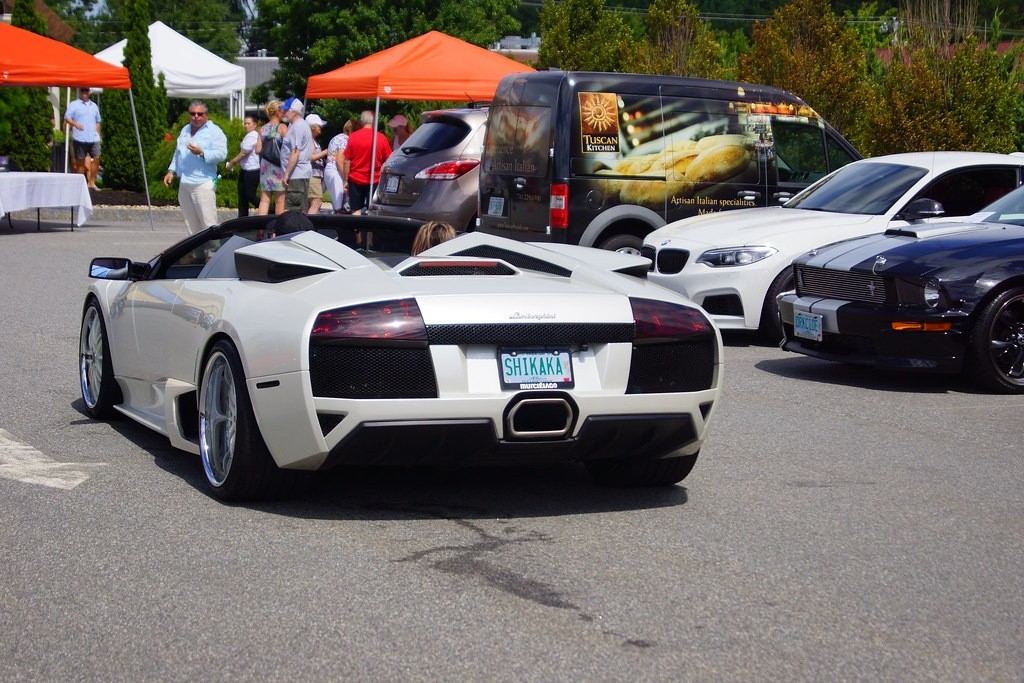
[369,103,491,236]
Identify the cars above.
[641,150,1024,344]
[774,186,1024,395]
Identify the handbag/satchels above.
[262,124,282,166]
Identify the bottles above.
[213,174,222,185]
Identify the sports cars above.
[78,213,724,504]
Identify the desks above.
[0,172,94,231]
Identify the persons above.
[278,97,312,214]
[343,110,393,252]
[225,114,261,218]
[266,208,316,238]
[164,101,227,264]
[305,114,327,214]
[411,222,456,257]
[387,115,413,152]
[324,120,362,215]
[65,87,102,191]
[255,100,287,243]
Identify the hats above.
[306,114,327,125]
[80,86,90,91]
[278,97,304,112]
[387,115,408,128]
[274,210,316,236]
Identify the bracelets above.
[168,169,175,174]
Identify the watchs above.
[198,151,203,157]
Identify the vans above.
[476,72,864,265]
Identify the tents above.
[0,21,154,231]
[304,30,539,213]
[93,21,246,127]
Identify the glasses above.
[189,112,203,116]
[394,128,397,133]
